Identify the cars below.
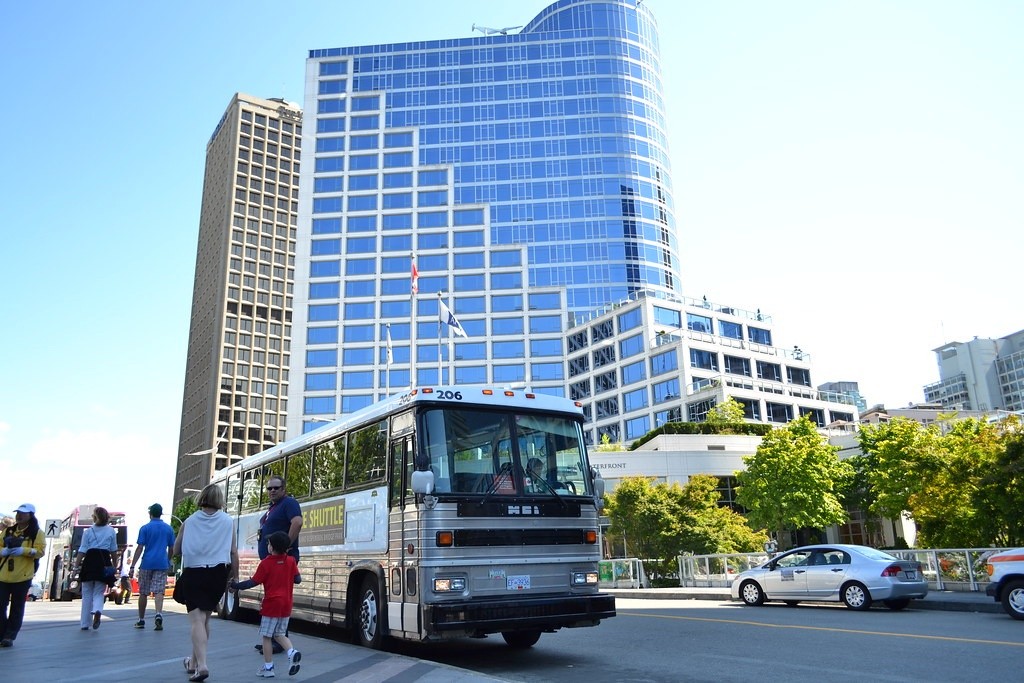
[729,543,929,611]
[26,580,43,602]
[985,546,1024,622]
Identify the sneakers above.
[256,663,275,677]
[154,613,163,630]
[288,649,302,675]
[133,619,145,627]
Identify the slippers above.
[183,657,195,673]
[189,668,209,681]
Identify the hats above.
[13,503,35,513]
[265,531,291,551]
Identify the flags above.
[439,296,468,341]
[411,257,419,297]
[386,327,393,364]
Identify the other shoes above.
[124,602,132,604]
[93,610,101,629]
[255,638,284,654]
[0,639,13,647]
[81,627,88,630]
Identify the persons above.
[228,531,302,678]
[119,557,132,604]
[129,504,176,630]
[524,457,545,494]
[173,485,239,681]
[72,508,117,630]
[0,504,46,647]
[254,476,303,655]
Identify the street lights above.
[147,488,202,576]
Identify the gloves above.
[228,577,239,593]
[1,547,9,556]
[11,546,24,557]
[172,554,181,573]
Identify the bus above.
[48,503,128,604]
[211,384,619,654]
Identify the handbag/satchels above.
[104,566,116,577]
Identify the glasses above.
[267,486,282,490]
[266,542,268,544]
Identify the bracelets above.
[73,567,79,570]
[130,566,134,569]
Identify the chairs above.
[830,555,839,562]
[816,554,826,562]
